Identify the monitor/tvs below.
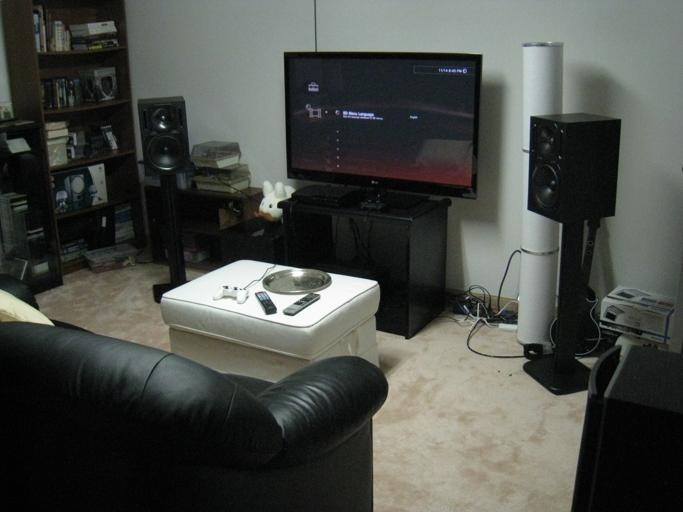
[283,52,483,209]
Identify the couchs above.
[0,275,388,511]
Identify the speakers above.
[138,95,189,304]
[525,113,621,356]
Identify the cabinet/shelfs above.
[278,197,452,339]
[145,184,264,272]
[0,119,63,294]
[0,0,148,274]
[571,345,682,511]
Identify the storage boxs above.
[85,244,138,273]
[601,284,677,345]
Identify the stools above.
[160,259,381,384]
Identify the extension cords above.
[498,322,518,330]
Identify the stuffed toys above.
[252,180,298,222]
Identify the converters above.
[453,301,469,314]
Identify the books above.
[41,67,82,109]
[59,237,89,264]
[33,3,119,54]
[44,121,72,139]
[112,203,136,244]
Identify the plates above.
[263,268,332,295]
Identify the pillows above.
[0,288,54,326]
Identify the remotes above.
[255,291,277,315]
[282,292,320,316]
